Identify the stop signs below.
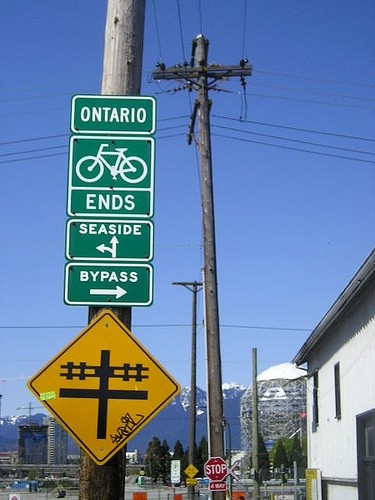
[204,457,228,483]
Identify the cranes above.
[16,402,46,424]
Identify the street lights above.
[185,405,233,500]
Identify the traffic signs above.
[64,261,154,308]
[66,218,154,263]
